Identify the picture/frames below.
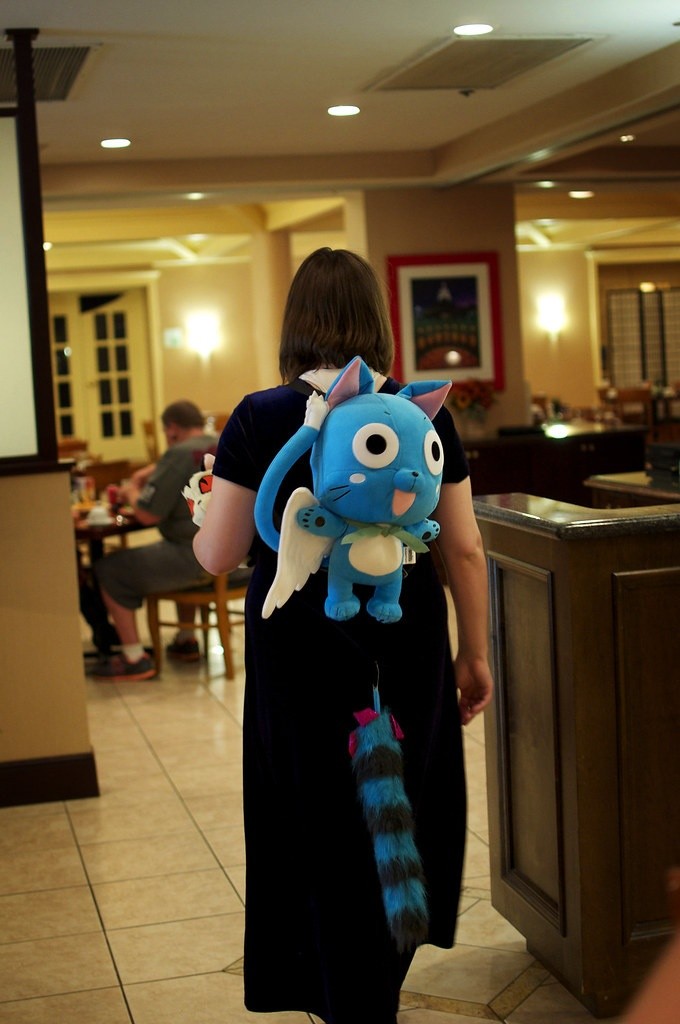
[384,247,506,396]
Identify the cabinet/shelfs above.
[461,421,650,511]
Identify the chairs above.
[58,414,256,681]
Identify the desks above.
[72,511,159,656]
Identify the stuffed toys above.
[181,454,253,569]
[349,709,428,952]
[255,357,453,623]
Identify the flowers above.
[447,372,495,430]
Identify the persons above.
[93,400,250,680]
[193,245,492,1024]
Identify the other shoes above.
[166,640,200,662]
[101,652,157,680]
